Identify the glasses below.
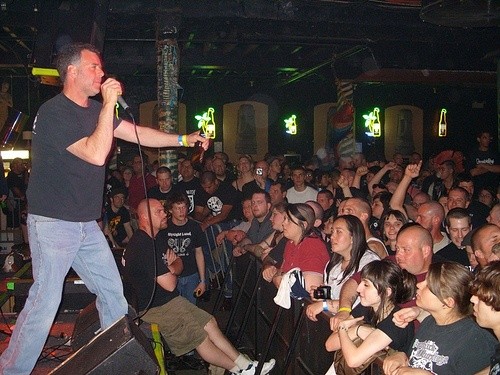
[305,174,312,177]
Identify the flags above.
[328,103,353,150]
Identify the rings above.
[308,315,312,317]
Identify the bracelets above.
[337,307,351,312]
[178,135,183,146]
[182,134,189,147]
[338,326,348,332]
[323,300,328,311]
[264,240,269,245]
[199,280,208,284]
[356,324,363,337]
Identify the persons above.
[120,198,276,375]
[0,132,500,375]
[0,44,209,375]
[0,82,13,130]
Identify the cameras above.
[314,286,331,299]
[256,168,262,175]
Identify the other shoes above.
[232,369,246,375]
[209,271,226,289]
[239,359,275,375]
[223,288,232,298]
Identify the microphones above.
[104,77,130,111]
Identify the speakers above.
[44,313,161,375]
[70,287,139,352]
